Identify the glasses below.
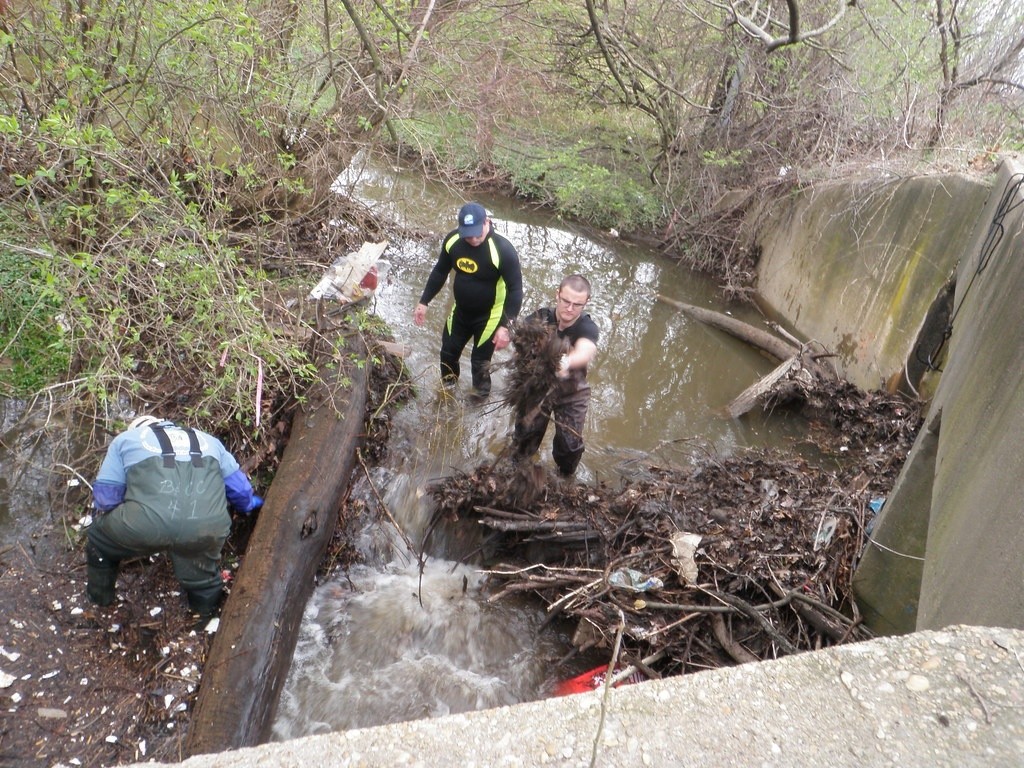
[559,292,588,310]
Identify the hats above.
[459,203,486,238]
[128,415,164,430]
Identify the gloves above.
[252,496,264,508]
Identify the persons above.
[413,203,522,409]
[84,414,264,635]
[511,274,599,479]
